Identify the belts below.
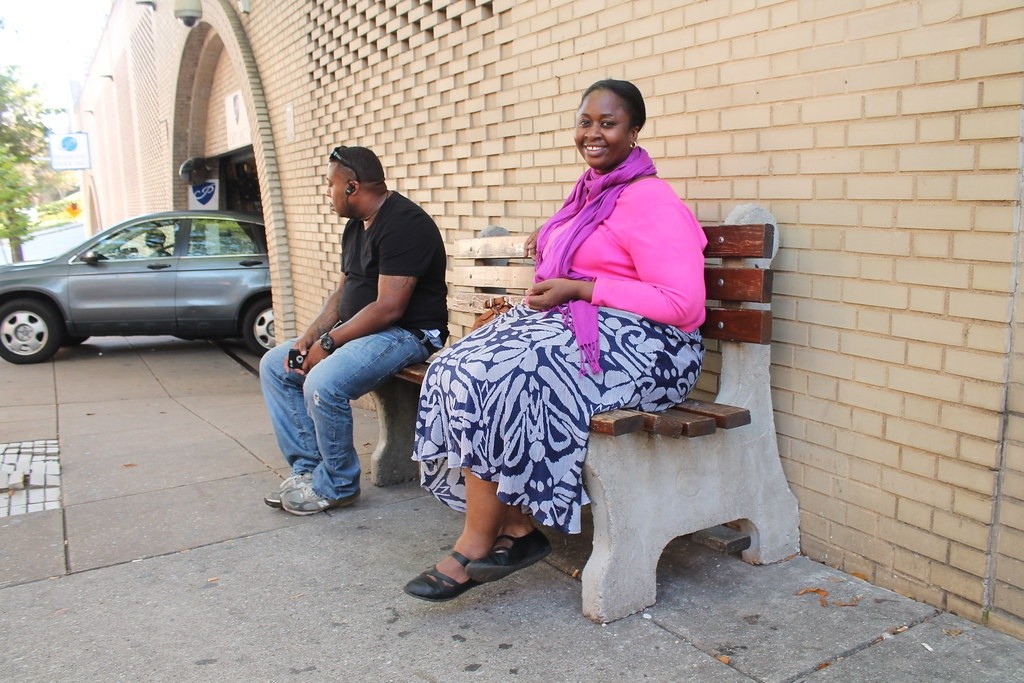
[405,328,437,355]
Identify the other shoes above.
[404,551,481,602]
[465,528,552,583]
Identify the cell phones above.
[288,349,309,369]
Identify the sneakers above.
[264,472,312,508]
[280,480,361,516]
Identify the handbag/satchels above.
[470,296,514,334]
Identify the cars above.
[0,209,275,366]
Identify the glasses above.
[329,146,360,183]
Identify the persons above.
[258,147,449,517]
[144,228,172,258]
[401,80,706,602]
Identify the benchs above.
[365,206,803,624]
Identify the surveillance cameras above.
[173,0,202,27]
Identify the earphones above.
[346,185,356,194]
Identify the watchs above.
[320,332,334,353]
